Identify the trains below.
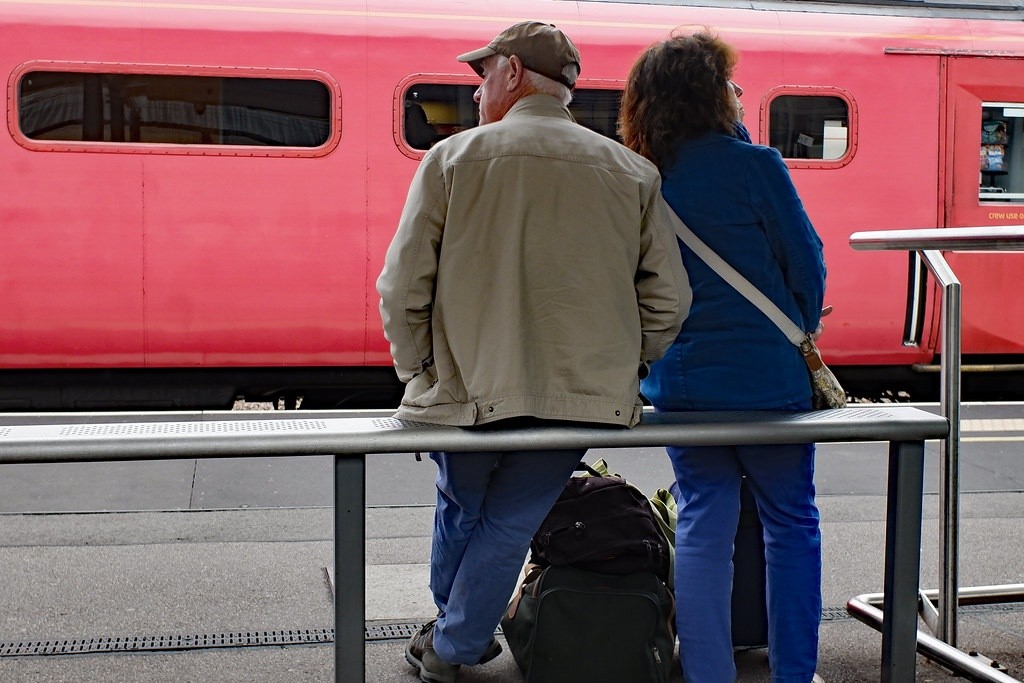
[0,2,1022,412]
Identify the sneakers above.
[480,634,503,663]
[404,619,461,682]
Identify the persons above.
[617,25,827,683]
[373,18,692,683]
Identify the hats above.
[456,20,582,92]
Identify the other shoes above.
[809,674,824,683]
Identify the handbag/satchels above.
[799,332,847,409]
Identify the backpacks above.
[500,463,676,682]
[640,479,769,648]
